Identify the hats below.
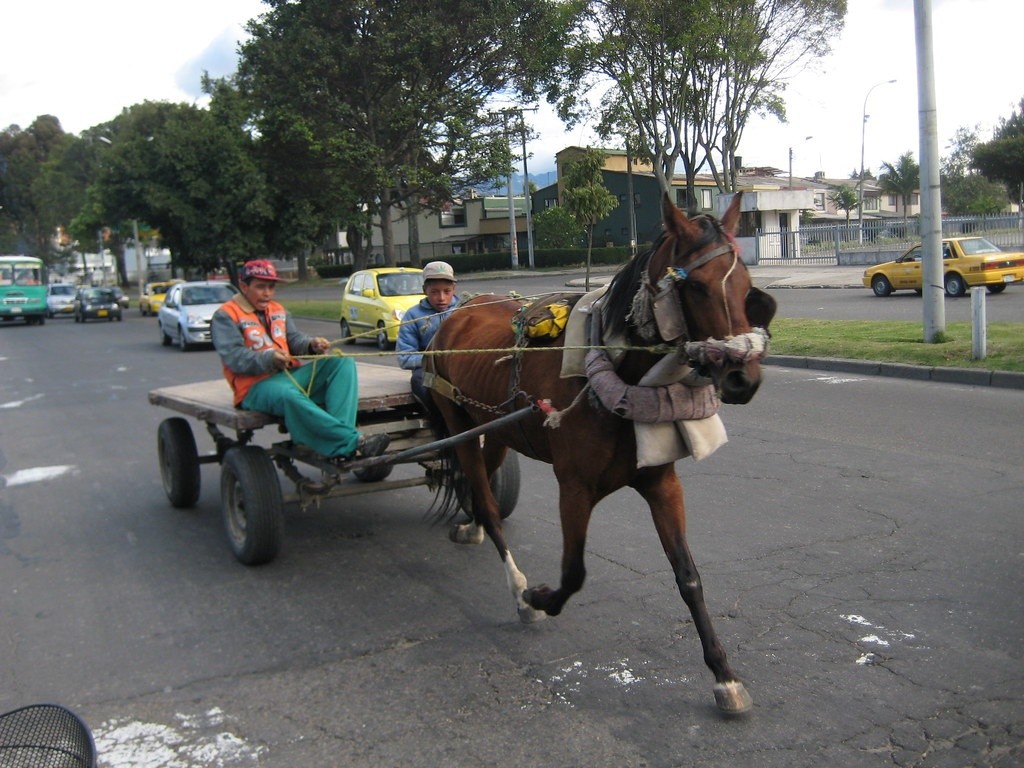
[423,261,457,284]
[242,260,282,281]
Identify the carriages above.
[148,193,777,714]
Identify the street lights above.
[789,136,813,190]
[859,80,898,243]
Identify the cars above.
[158,281,241,350]
[140,281,181,316]
[46,284,77,318]
[75,285,130,323]
[340,267,429,350]
[862,236,1024,297]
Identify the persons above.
[210,259,390,458]
[396,261,463,433]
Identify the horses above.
[413,185,776,718]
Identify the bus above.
[0,256,48,325]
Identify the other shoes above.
[356,433,390,460]
